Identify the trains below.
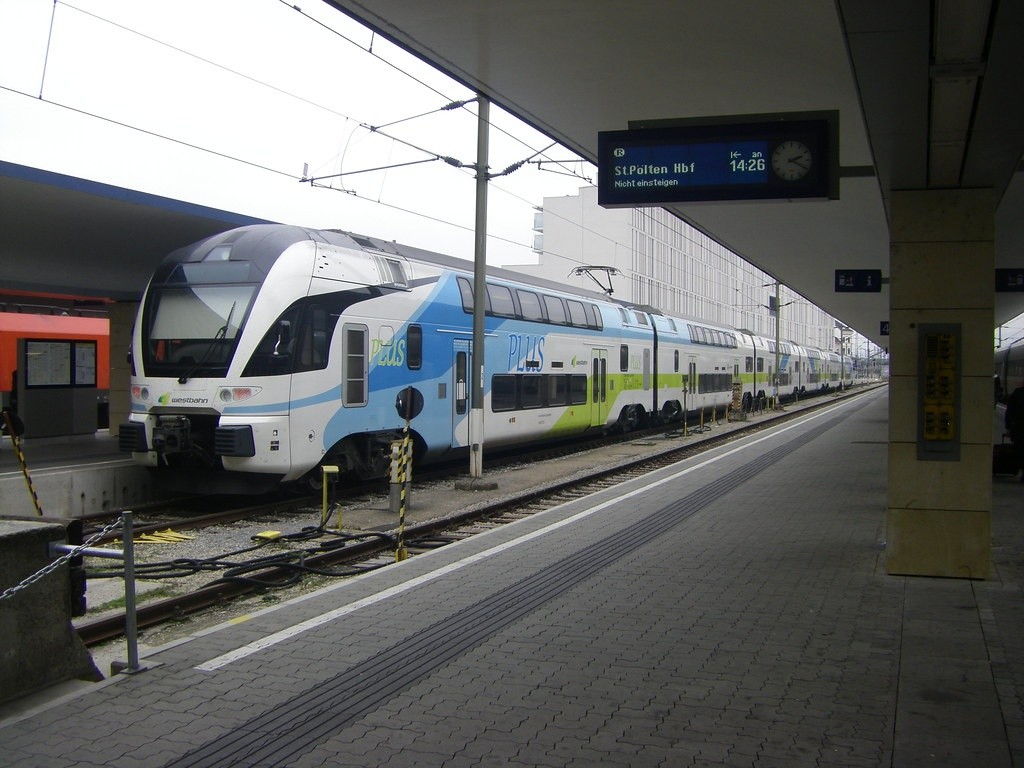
[0,312,109,435]
[994,343,1024,404]
[118,223,887,492]
